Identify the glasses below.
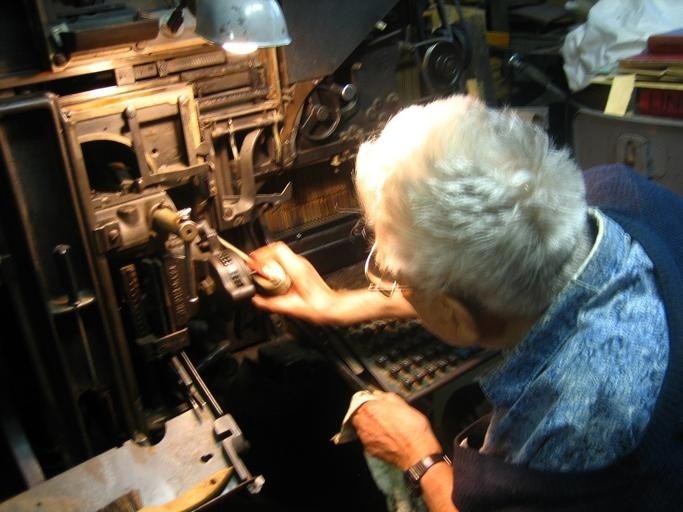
[362,243,430,299]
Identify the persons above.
[245,88,683,511]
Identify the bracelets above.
[402,450,453,498]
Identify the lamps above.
[194,0,293,54]
